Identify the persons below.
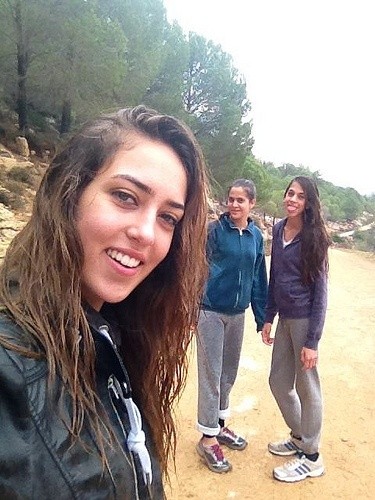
[261,175,330,484]
[0,102,212,500]
[195,178,267,474]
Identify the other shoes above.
[273,452,326,483]
[196,436,232,472]
[268,438,303,456]
[217,423,248,450]
